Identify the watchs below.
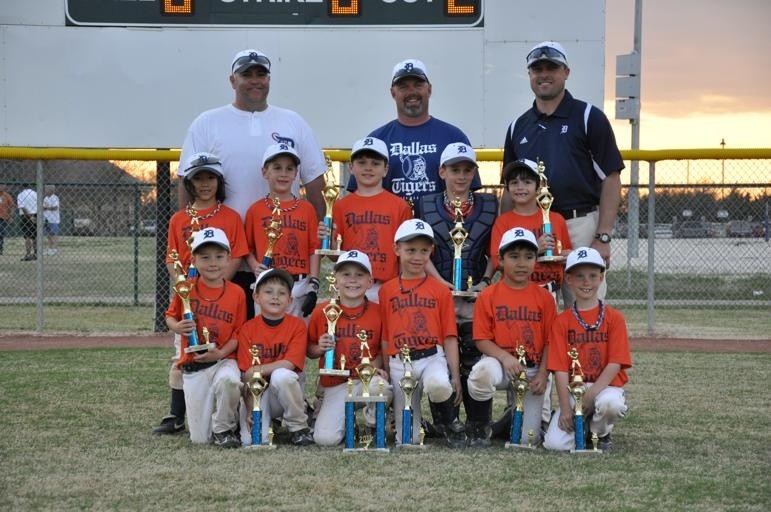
[594,233,611,243]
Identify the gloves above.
[302,277,320,317]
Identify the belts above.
[561,203,599,220]
[291,273,307,282]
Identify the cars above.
[72,213,95,236]
[128,218,157,236]
[618,222,653,240]
[672,220,714,237]
[722,215,762,237]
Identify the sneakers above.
[431,421,492,448]
[212,431,240,448]
[598,432,613,456]
[291,429,314,446]
[154,414,185,434]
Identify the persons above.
[0,182,60,262]
[166,137,572,435]
[542,246,632,452]
[499,42,625,309]
[236,268,315,446]
[344,59,481,217]
[177,49,328,317]
[378,218,468,445]
[306,250,394,447]
[462,226,557,448]
[162,226,247,447]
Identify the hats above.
[565,246,606,273]
[394,218,434,243]
[191,226,229,254]
[439,142,478,168]
[498,227,538,250]
[502,158,539,185]
[260,143,300,168]
[349,137,389,162]
[183,152,223,179]
[391,58,428,85]
[231,49,270,73]
[334,249,373,277]
[525,41,569,68]
[255,268,295,293]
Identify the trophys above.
[504,338,538,451]
[343,330,391,454]
[398,344,429,450]
[568,347,603,452]
[247,344,277,451]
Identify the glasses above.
[526,46,567,66]
[231,55,270,72]
[392,68,428,85]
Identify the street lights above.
[719,138,728,196]
